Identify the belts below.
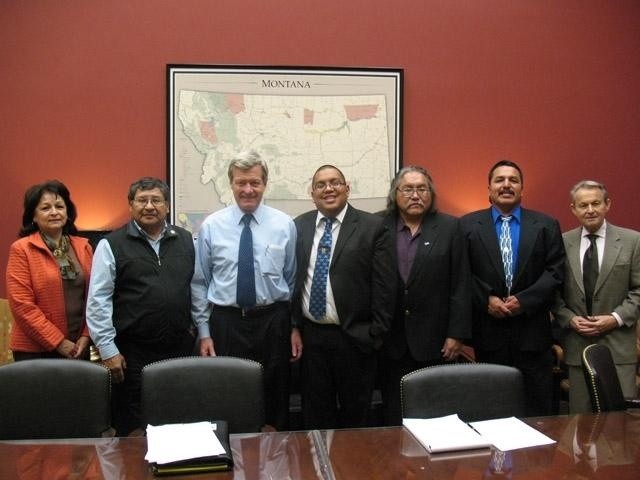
[213,300,285,317]
[300,314,342,333]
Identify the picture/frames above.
[163,61,406,251]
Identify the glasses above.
[313,181,346,192]
[129,197,169,208]
[396,185,431,197]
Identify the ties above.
[235,212,260,313]
[579,233,602,317]
[307,217,336,320]
[497,214,516,296]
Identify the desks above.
[1,407,639,480]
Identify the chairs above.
[580,341,640,415]
[400,362,527,425]
[0,358,115,441]
[139,354,266,438]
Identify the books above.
[400,413,491,454]
[153,419,233,477]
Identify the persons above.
[457,161,567,416]
[369,167,474,426]
[6,179,96,359]
[294,165,398,430]
[190,151,303,432]
[86,177,196,437]
[547,181,639,415]
